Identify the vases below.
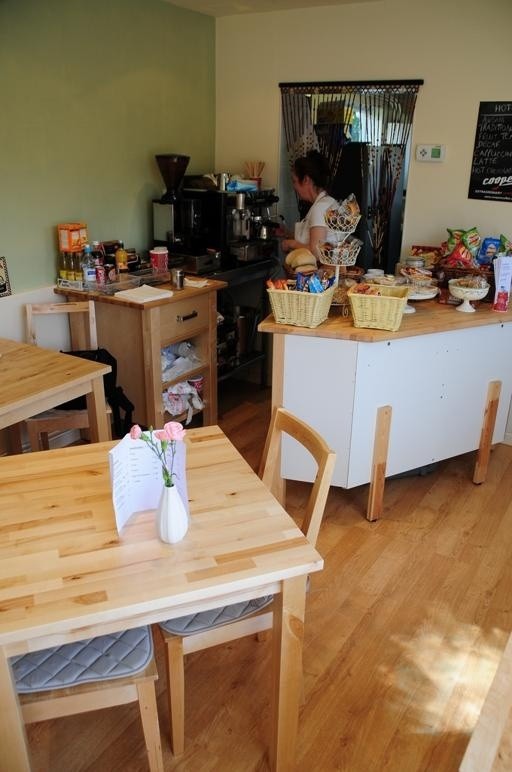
[154,492,191,545]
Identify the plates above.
[397,289,438,302]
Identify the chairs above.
[152,404,337,756]
[0,622,164,772]
[24,300,113,451]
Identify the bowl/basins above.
[368,269,384,274]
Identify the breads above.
[285,200,361,275]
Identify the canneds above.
[95,266,107,289]
[105,264,117,284]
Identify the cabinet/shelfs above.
[54,271,230,431]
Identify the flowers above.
[126,420,187,488]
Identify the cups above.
[187,375,205,398]
[170,266,183,291]
[150,250,167,275]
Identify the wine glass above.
[448,282,491,315]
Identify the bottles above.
[57,240,127,290]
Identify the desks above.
[207,257,278,418]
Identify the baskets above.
[347,283,412,333]
[266,275,336,330]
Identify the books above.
[112,282,174,305]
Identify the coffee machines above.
[152,152,286,274]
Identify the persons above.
[272,147,348,271]
